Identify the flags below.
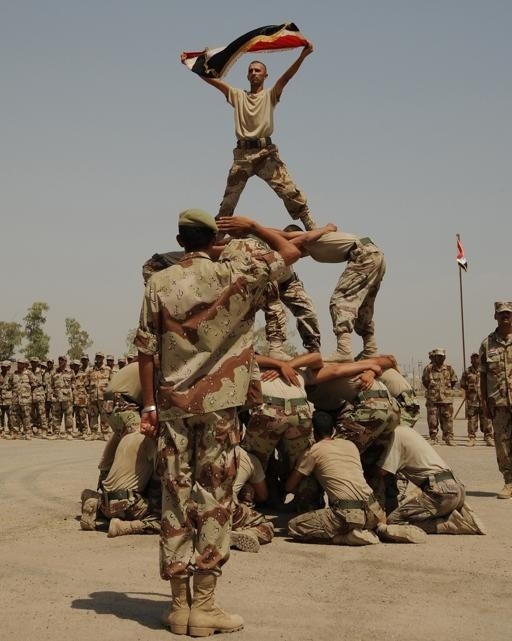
[184,22,307,77]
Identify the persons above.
[479,293,511,499]
[421,346,495,449]
[74,208,488,544]
[1,354,155,444]
[132,208,301,635]
[180,44,317,233]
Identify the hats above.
[429,302,512,360]
[0,352,138,367]
[179,209,219,234]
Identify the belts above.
[279,272,297,289]
[262,396,306,406]
[238,137,271,151]
[420,470,453,491]
[332,492,378,509]
[398,392,415,402]
[349,237,372,253]
[355,391,388,403]
[102,393,114,400]
[153,254,169,270]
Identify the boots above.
[159,576,190,633]
[332,506,485,544]
[269,333,380,369]
[1,428,112,440]
[230,528,260,553]
[430,436,495,446]
[498,484,512,499]
[80,489,144,537]
[299,211,321,231]
[190,571,243,636]
